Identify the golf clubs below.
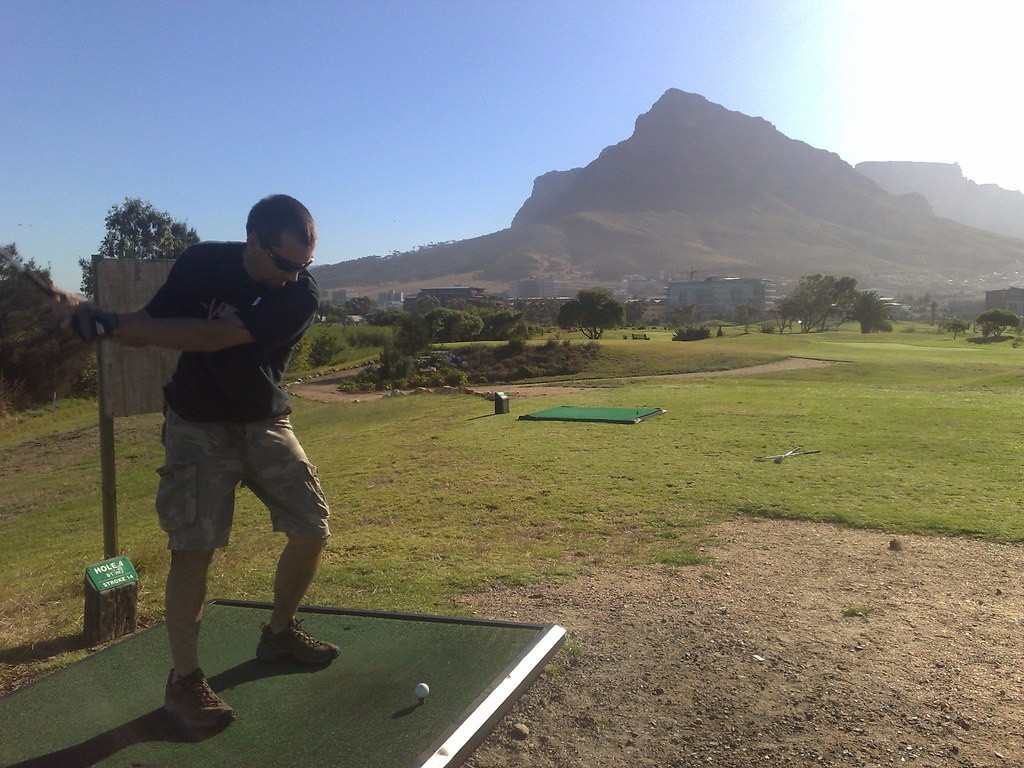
[0,247,106,339]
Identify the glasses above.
[258,237,314,272]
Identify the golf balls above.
[415,682,429,698]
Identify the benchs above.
[632,334,649,340]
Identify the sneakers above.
[255,618,340,665]
[164,668,235,729]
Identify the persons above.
[34,193,340,727]
